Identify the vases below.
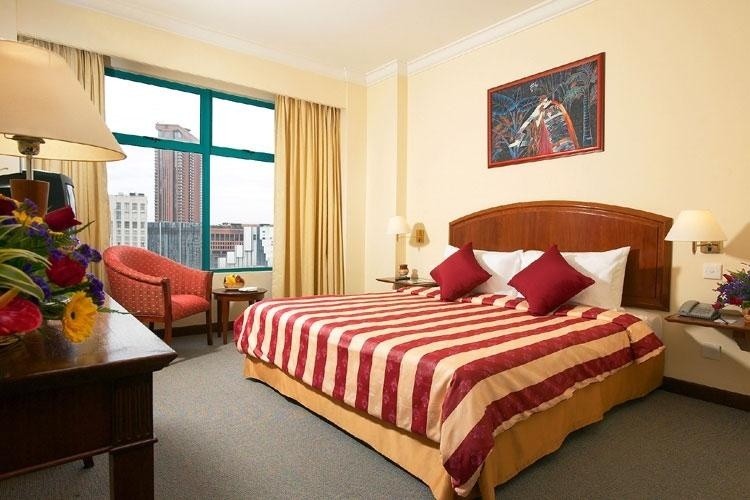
[742,308,750,326]
[0,331,26,346]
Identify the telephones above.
[676,300,721,320]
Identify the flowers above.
[0,197,105,345]
[712,262,750,311]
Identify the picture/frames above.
[486,52,607,168]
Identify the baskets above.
[224,282,244,288]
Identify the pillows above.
[430,243,631,317]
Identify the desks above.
[0,289,177,500]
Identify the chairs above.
[103,244,214,347]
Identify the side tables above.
[212,287,267,345]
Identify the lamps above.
[1,39,126,218]
[663,210,728,256]
[385,216,424,244]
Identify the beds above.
[231,200,672,500]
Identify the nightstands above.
[375,276,438,287]
[664,308,750,333]
